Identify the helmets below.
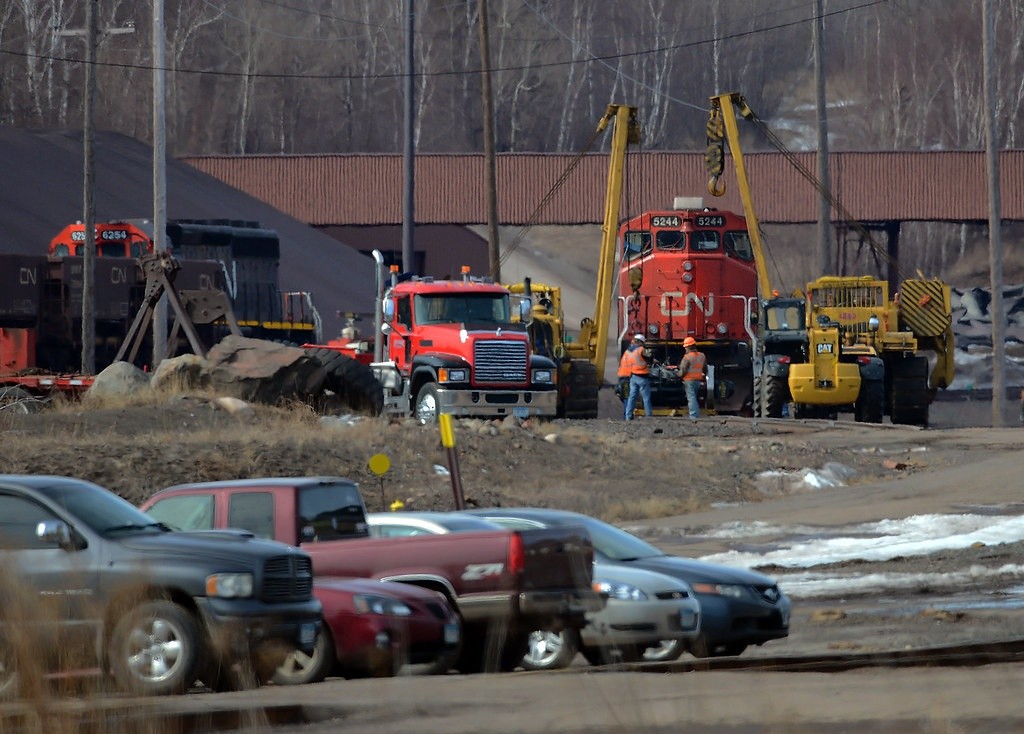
[683,337,695,347]
[634,334,645,342]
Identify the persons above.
[617,333,654,422]
[672,336,707,422]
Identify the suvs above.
[0,470,323,699]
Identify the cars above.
[310,572,461,686]
[358,504,792,671]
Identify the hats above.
[533,305,547,310]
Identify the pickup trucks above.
[138,474,594,675]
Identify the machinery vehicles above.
[0,218,385,419]
[486,101,640,423]
[701,87,957,426]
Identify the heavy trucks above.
[616,205,763,416]
[299,247,560,424]
